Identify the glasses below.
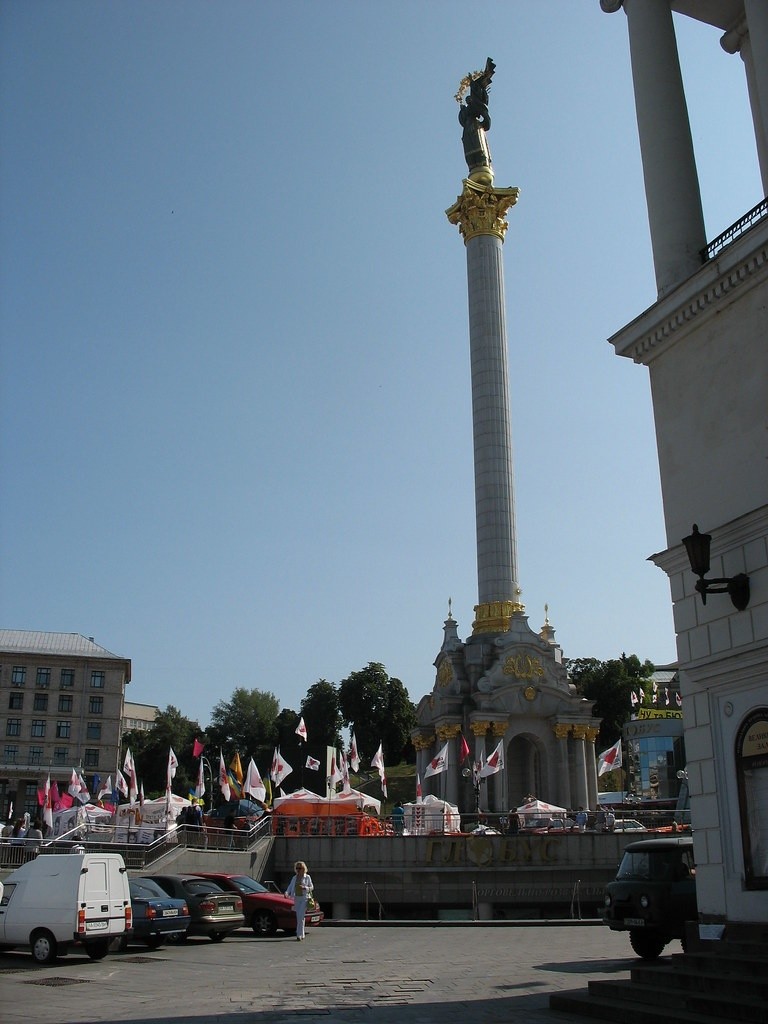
[296,868,303,870]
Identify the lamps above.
[680,524,752,611]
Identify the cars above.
[108,876,192,956]
[141,875,246,946]
[175,872,325,938]
[518,816,649,835]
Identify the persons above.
[0,806,53,862]
[67,818,74,836]
[386,802,405,836]
[176,796,271,850]
[284,861,315,940]
[593,803,615,831]
[459,97,492,170]
[571,807,589,831]
[53,817,61,837]
[508,808,521,834]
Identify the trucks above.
[598,837,697,962]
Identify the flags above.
[459,734,504,790]
[416,775,422,804]
[295,717,387,798]
[424,743,448,779]
[598,738,622,777]
[36,740,294,827]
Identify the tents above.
[509,800,567,826]
[403,795,460,831]
[46,794,192,843]
[273,787,381,828]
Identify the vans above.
[0,853,134,965]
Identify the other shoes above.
[297,937,301,941]
[301,937,304,940]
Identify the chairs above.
[665,861,689,881]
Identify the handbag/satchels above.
[307,887,314,909]
